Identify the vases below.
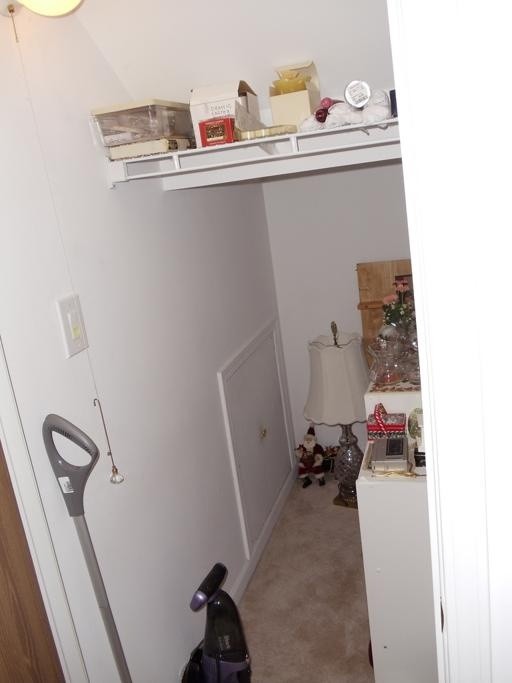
[366,322,421,384]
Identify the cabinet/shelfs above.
[356,382,438,682]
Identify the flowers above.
[381,280,417,340]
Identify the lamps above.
[303,321,371,509]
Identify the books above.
[109,136,199,159]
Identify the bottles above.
[366,325,421,386]
[317,98,345,124]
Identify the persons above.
[293,422,331,487]
